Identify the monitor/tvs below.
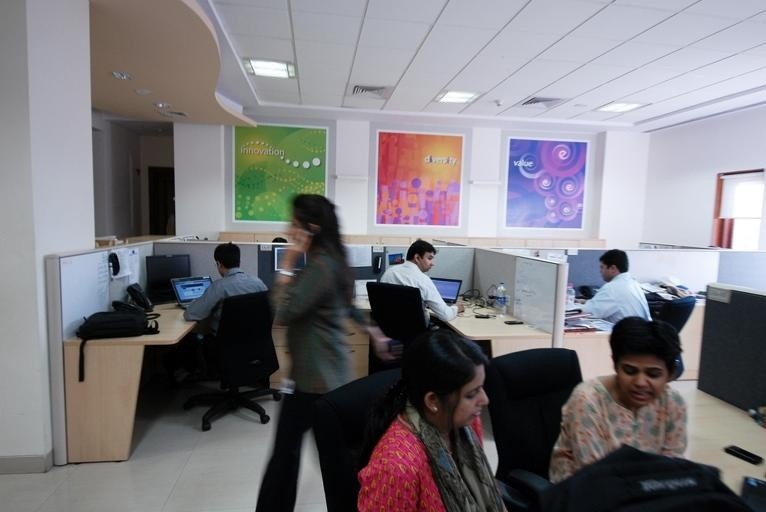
[275,248,306,272]
[146,254,190,304]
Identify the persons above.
[379,239,464,328]
[271,237,287,242]
[184,242,269,336]
[254,193,394,511]
[357,328,509,512]
[549,315,688,487]
[574,249,653,323]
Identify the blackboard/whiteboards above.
[510,256,560,335]
[58,248,113,341]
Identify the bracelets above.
[277,269,296,278]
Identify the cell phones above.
[725,445,762,464]
[475,314,490,318]
[505,320,523,325]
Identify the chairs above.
[481,345,585,510]
[176,287,283,432]
[655,296,699,334]
[304,362,411,510]
[364,277,442,346]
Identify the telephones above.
[127,282,154,309]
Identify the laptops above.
[430,277,462,306]
[170,275,213,309]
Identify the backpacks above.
[75,309,159,339]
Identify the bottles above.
[566,283,576,306]
[497,283,507,317]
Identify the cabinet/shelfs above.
[264,320,298,396]
[342,308,372,377]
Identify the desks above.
[668,385,766,498]
[61,299,203,466]
[565,296,708,384]
[347,291,553,358]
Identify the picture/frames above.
[504,135,591,233]
[373,128,465,228]
[230,121,329,225]
